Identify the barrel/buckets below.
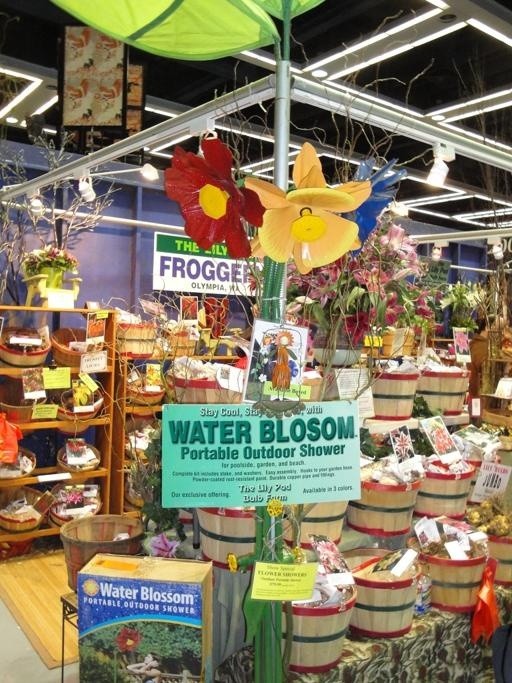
[481,525,512,587]
[279,501,347,552]
[350,462,412,533]
[417,457,475,518]
[337,549,416,639]
[279,569,359,671]
[60,514,143,593]
[365,366,418,421]
[419,368,471,418]
[0,317,246,528]
[192,503,273,574]
[409,530,487,613]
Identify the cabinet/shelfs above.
[110,351,240,520]
[2,302,118,543]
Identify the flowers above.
[157,132,487,361]
